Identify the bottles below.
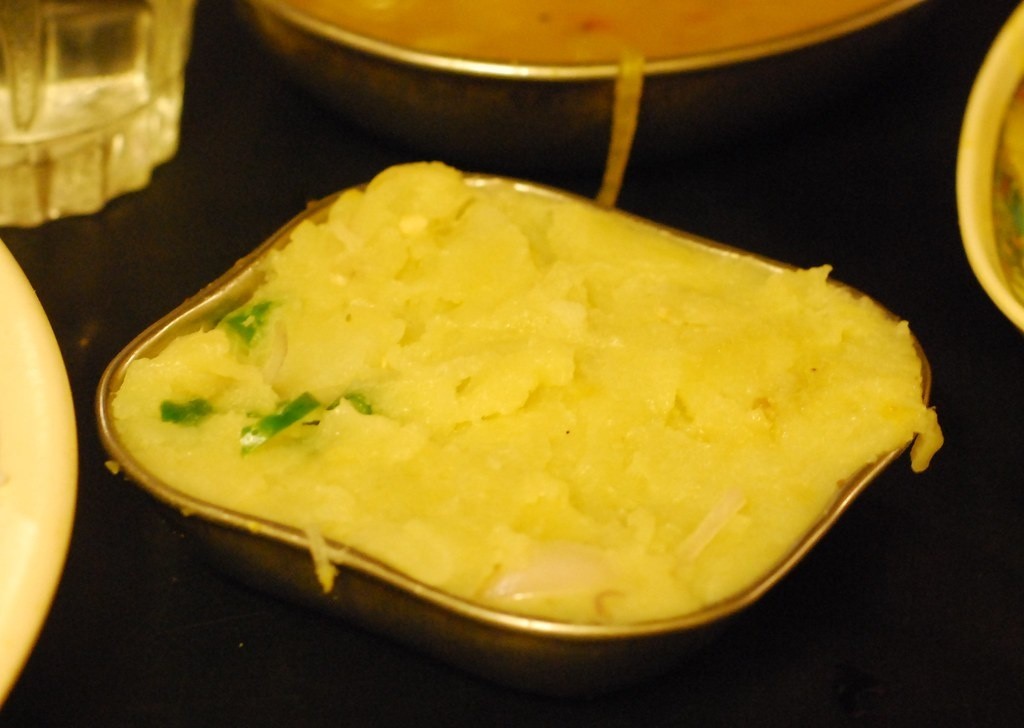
[0,0,189,227]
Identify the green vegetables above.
[160,302,373,453]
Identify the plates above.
[93,159,932,687]
[0,236,80,712]
[208,0,925,178]
[958,15,1023,326]
